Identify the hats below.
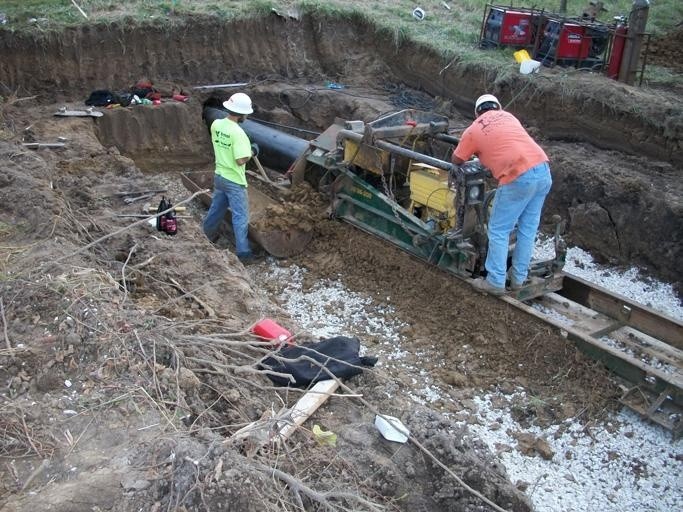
[223,92,255,115]
[474,94,502,120]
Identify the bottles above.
[141,99,153,105]
[156,195,177,236]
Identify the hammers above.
[143,202,187,214]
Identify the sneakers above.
[238,251,266,266]
[507,265,523,291]
[204,229,222,243]
[469,277,507,296]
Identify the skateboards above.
[54,106,103,118]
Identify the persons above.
[449,93,551,298]
[200,92,267,259]
